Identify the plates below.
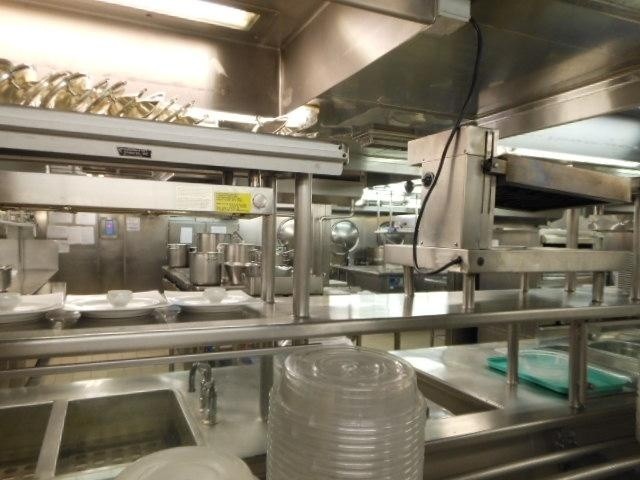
[0,289,260,323]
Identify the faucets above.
[187,361,216,425]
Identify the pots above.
[167,232,261,285]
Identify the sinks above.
[414,367,503,421]
[1,401,53,479]
[590,340,640,361]
[53,386,206,480]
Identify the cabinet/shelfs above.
[0,105,640,476]
